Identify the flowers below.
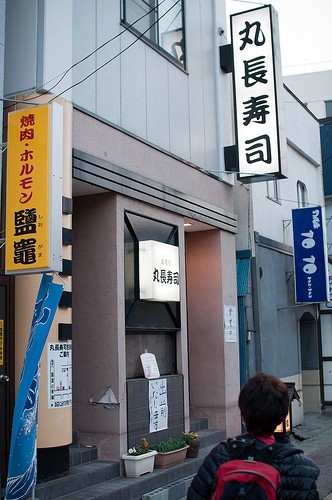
[183,430,201,443]
[129,436,149,456]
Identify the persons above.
[187,372,320,500]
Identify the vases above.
[121,450,157,476]
[186,440,200,457]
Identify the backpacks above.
[209,439,283,500]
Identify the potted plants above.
[148,437,189,467]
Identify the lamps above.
[274,383,300,444]
